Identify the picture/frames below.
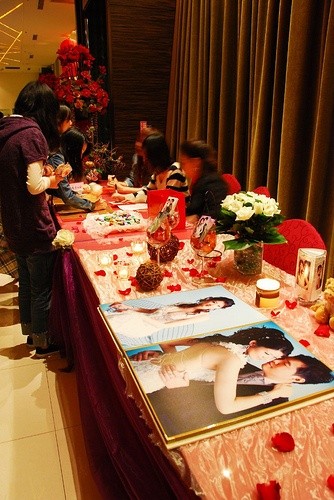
[124,320,334,449]
[99,284,269,353]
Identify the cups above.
[255,278,281,309]
[130,237,147,255]
[115,262,132,280]
[96,250,113,268]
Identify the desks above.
[52,196,334,500]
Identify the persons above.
[108,127,190,203]
[178,138,236,232]
[57,105,73,132]
[0,81,64,356]
[106,296,235,338]
[194,217,209,237]
[297,260,310,289]
[164,199,175,212]
[129,327,334,436]
[315,261,325,289]
[44,127,107,211]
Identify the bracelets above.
[259,390,272,404]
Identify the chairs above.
[263,219,326,275]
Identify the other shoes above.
[27,336,36,349]
[36,342,66,354]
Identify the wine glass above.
[146,215,170,274]
[189,222,217,284]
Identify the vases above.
[234,235,262,276]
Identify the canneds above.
[255,278,280,308]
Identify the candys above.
[95,212,141,226]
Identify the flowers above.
[81,126,126,181]
[39,37,109,113]
[220,190,287,251]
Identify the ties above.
[237,373,265,385]
